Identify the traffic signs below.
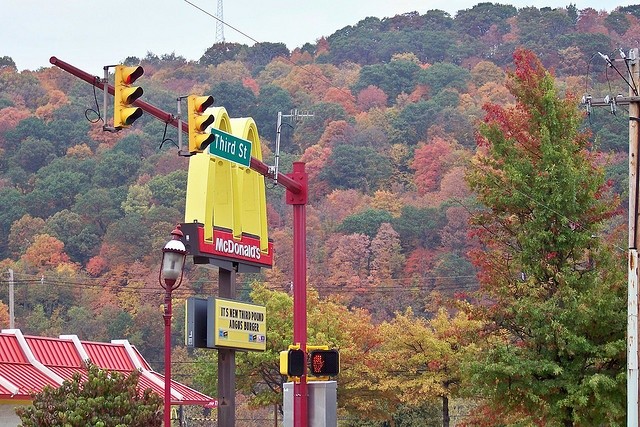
[208,126,252,167]
[206,297,266,351]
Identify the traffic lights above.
[279,341,303,380]
[187,93,214,152]
[306,345,339,380]
[113,64,143,127]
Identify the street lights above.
[159,224,186,426]
